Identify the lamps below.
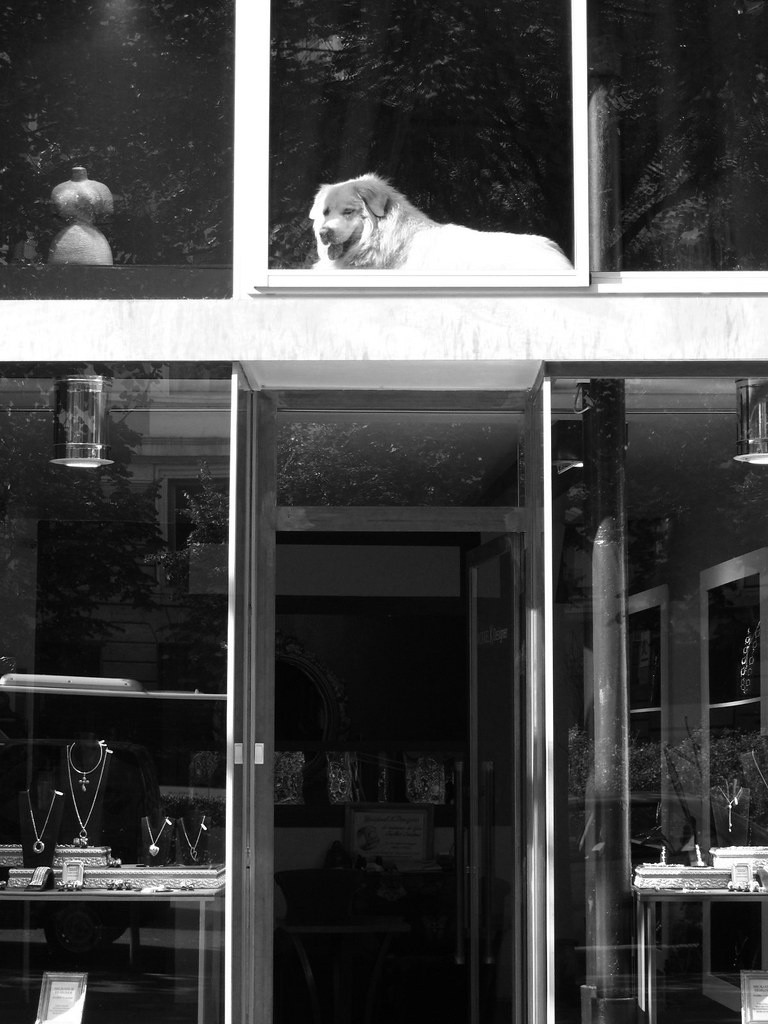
[48,378,114,468]
[732,377,768,465]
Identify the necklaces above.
[145,816,170,856]
[66,741,108,838]
[179,814,206,861]
[27,786,57,854]
[751,749,768,788]
[718,778,743,832]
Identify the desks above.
[1,884,225,1024]
[276,869,456,958]
[628,883,768,1024]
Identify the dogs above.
[307,172,575,270]
